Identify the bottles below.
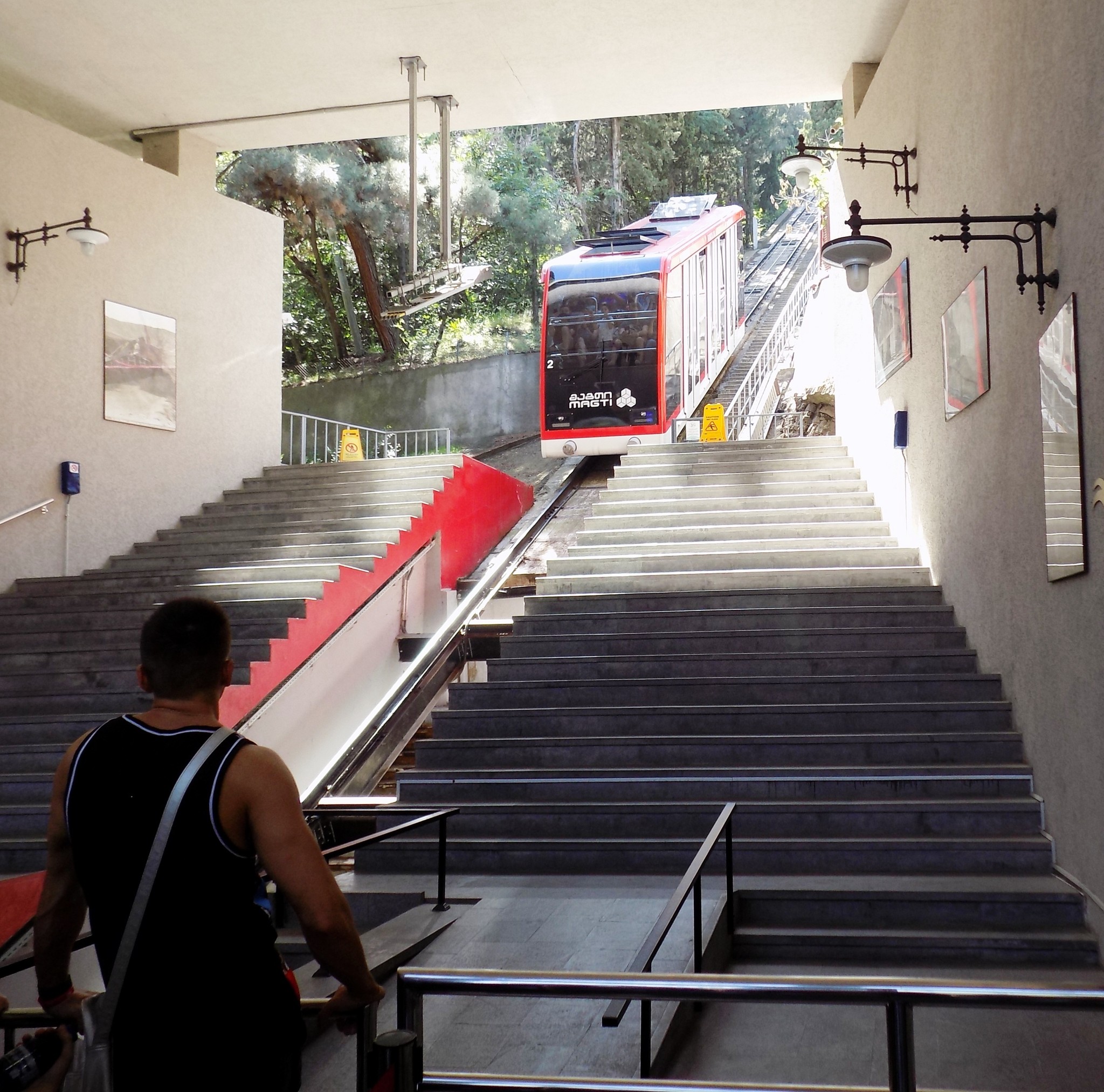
[0,1028,63,1092]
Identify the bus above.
[539,193,746,459]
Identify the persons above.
[545,285,660,367]
[31,592,386,1092]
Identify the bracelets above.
[38,974,74,1010]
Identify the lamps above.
[820,199,1059,315]
[781,134,918,208]
[6,207,109,283]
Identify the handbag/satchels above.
[64,991,113,1092]
